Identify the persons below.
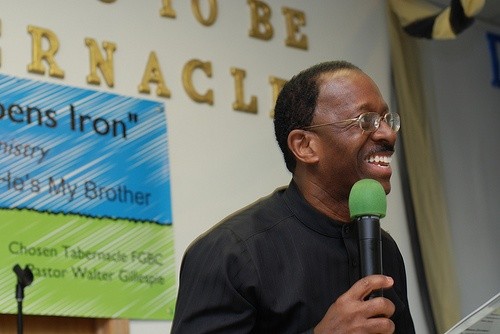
[171,61,416,334]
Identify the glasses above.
[302,112,400,132]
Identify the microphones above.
[349,179,387,318]
